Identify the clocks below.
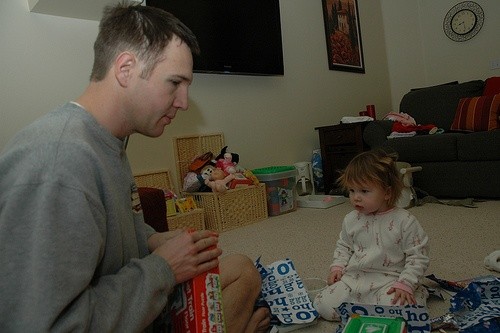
[443,1,484,42]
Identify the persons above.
[217,153,240,174]
[204,168,234,193]
[313,149,430,321]
[0,0,272,333]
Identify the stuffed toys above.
[484,249,500,272]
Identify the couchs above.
[364,76,500,200]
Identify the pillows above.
[455,94,499,132]
[484,77,500,96]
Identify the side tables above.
[316,118,379,197]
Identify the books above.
[164,186,196,216]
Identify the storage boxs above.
[343,314,407,333]
[161,227,227,333]
[253,164,300,215]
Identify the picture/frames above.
[321,0,366,74]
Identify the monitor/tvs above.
[145,0,285,77]
